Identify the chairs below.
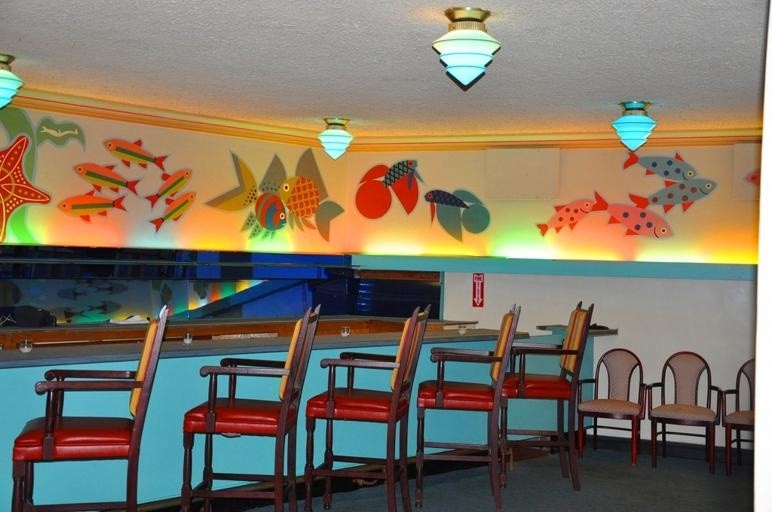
[723,359,756,477]
[10,304,171,511]
[501,301,594,490]
[304,304,434,511]
[416,304,522,512]
[182,304,321,512]
[648,351,723,474]
[577,348,648,467]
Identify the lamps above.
[430,8,501,88]
[610,101,657,152]
[317,117,353,161]
[0,54,24,110]
